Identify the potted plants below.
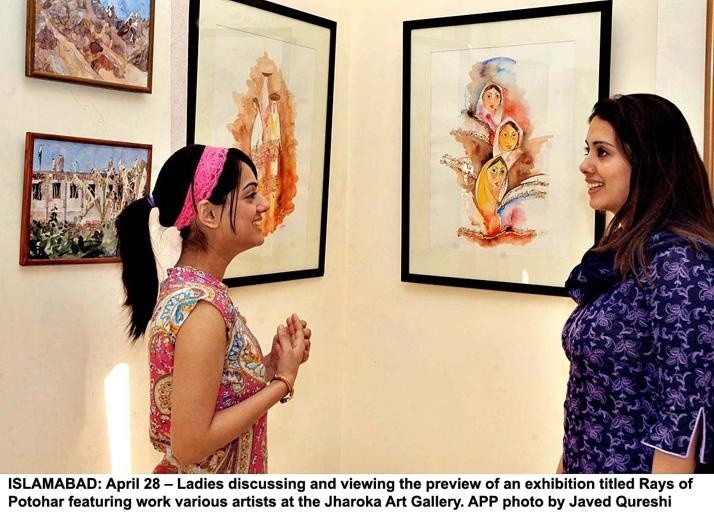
[400,0,613,298]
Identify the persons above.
[113,146,311,475]
[556,88,714,474]
[480,83,521,191]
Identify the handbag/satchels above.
[270,373,294,404]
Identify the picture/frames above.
[185,0,338,285]
[20,131,154,267]
[400,0,613,298]
[26,2,155,94]
